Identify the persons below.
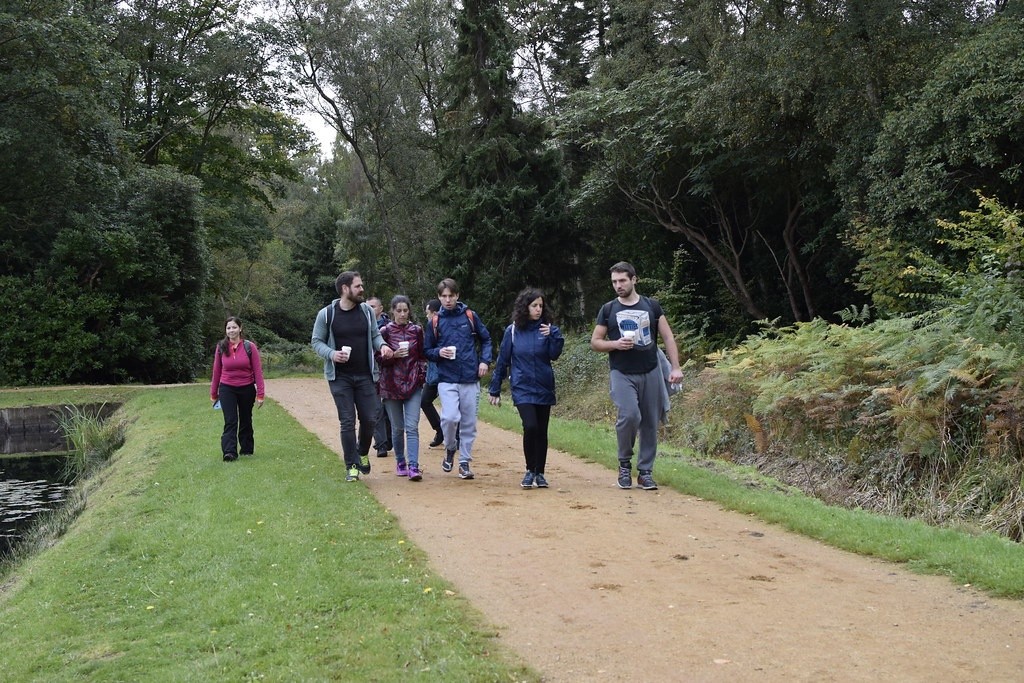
[375,296,427,480]
[311,271,393,483]
[209,317,265,461]
[420,298,460,451]
[422,277,492,479]
[489,287,564,491]
[363,295,393,456]
[591,261,684,489]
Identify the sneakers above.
[397,461,408,476]
[408,467,422,481]
[358,455,371,475]
[533,474,549,488]
[442,448,455,472]
[458,463,474,479]
[345,464,359,482]
[617,463,632,488]
[636,472,658,489]
[520,469,534,487]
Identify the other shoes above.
[224,454,235,461]
[456,440,461,450]
[377,447,387,457]
[430,432,444,447]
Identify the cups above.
[623,330,636,346]
[446,345,457,359]
[398,341,410,356]
[341,345,352,361]
